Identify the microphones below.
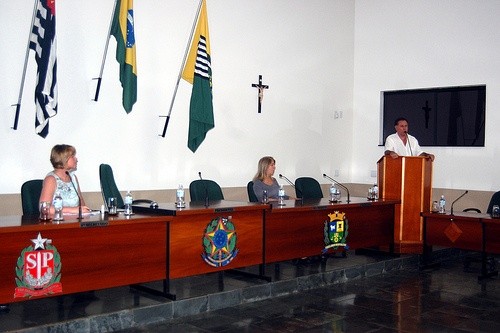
[403,130,413,155]
[279,174,304,205]
[65,171,83,220]
[199,172,209,207]
[450,190,468,216]
[323,173,350,202]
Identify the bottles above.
[176,184,185,207]
[124,190,132,215]
[439,196,446,213]
[53,192,63,220]
[373,185,379,200]
[329,184,336,201]
[278,185,285,205]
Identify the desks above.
[0,211,174,306]
[228,197,401,282]
[123,200,272,300]
[420,210,500,294]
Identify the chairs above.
[487,191,500,214]
[99,164,153,210]
[295,178,323,199]
[189,180,225,201]
[20,179,43,218]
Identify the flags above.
[29,0,58,139]
[181,0,214,153]
[110,0,137,114]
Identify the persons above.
[253,157,286,203]
[39,144,91,217]
[385,118,435,162]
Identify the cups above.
[432,201,438,211]
[336,189,341,200]
[492,205,500,217]
[108,197,117,215]
[39,202,51,222]
[368,188,373,199]
